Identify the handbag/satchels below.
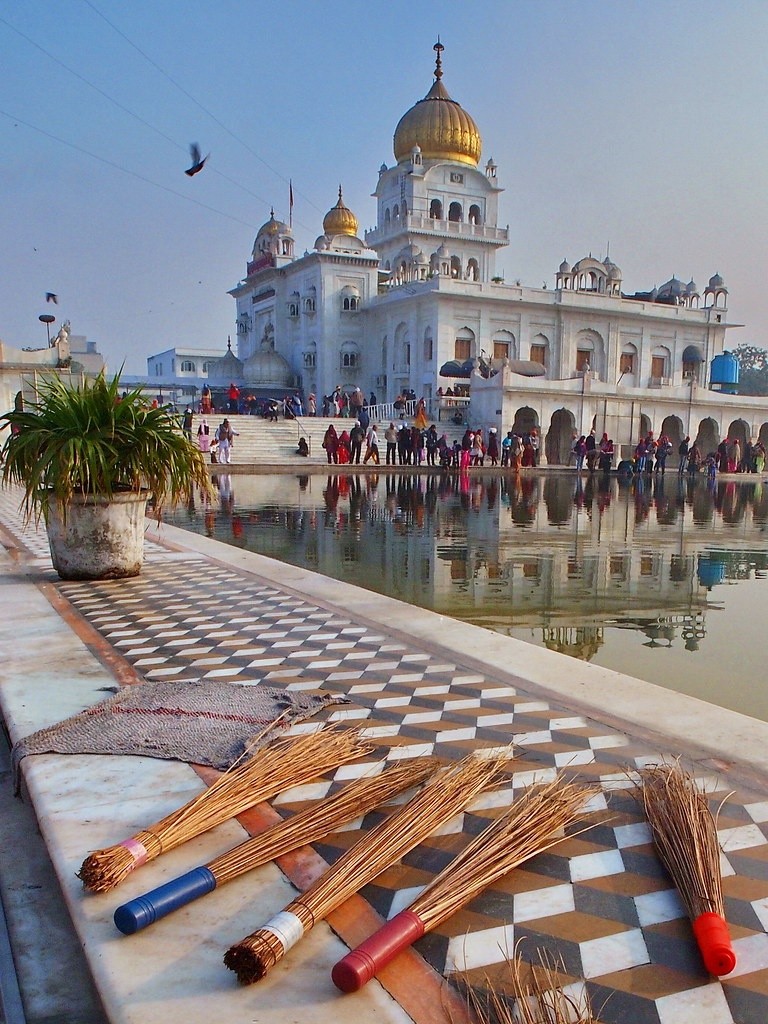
[481,445,487,454]
[419,446,427,462]
[322,442,327,448]
[478,448,484,458]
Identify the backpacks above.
[393,400,404,409]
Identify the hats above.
[723,438,728,443]
[639,429,659,447]
[224,418,229,423]
[401,421,407,427]
[397,424,403,430]
[530,430,538,436]
[231,383,236,387]
[733,438,739,443]
[355,388,361,392]
[355,421,361,426]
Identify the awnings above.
[441,357,546,377]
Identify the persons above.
[188,473,538,539]
[565,429,766,477]
[10,410,27,469]
[151,384,539,469]
[0,444,4,464]
[575,476,763,518]
[116,392,128,404]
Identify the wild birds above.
[184,140,211,179]
[45,291,59,305]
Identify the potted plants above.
[0,360,213,580]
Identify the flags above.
[290,184,294,206]
